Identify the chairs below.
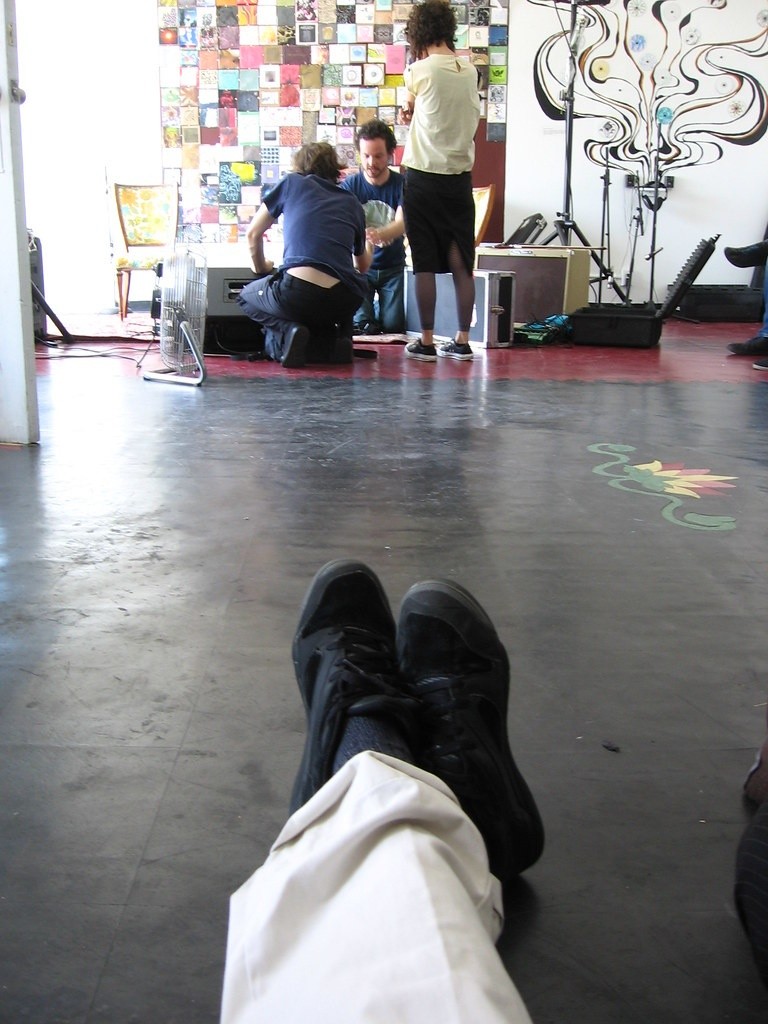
[113,184,173,323]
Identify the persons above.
[236,142,373,368]
[339,120,406,334]
[397,0,480,362]
[219,558,546,1023]
[733,736,768,988]
[724,222,768,371]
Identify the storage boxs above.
[568,234,720,346]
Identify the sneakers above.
[404,339,437,361]
[437,336,474,361]
[288,559,397,819]
[395,577,544,879]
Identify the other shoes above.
[724,239,768,268]
[727,337,768,355]
[752,358,768,370]
[328,336,353,365]
[281,323,310,367]
